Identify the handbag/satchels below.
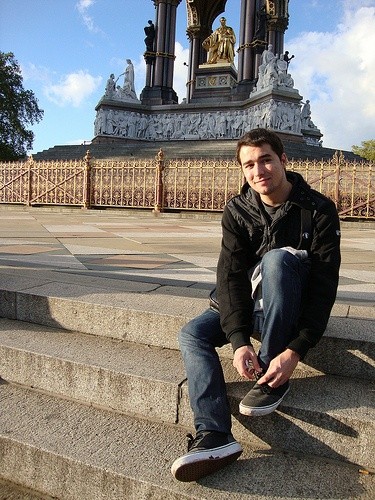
[209,288,220,314]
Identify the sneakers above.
[239,370,290,417]
[171,431,243,482]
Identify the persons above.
[201,17,236,64]
[94,43,323,146]
[144,20,156,52]
[170,128,341,482]
